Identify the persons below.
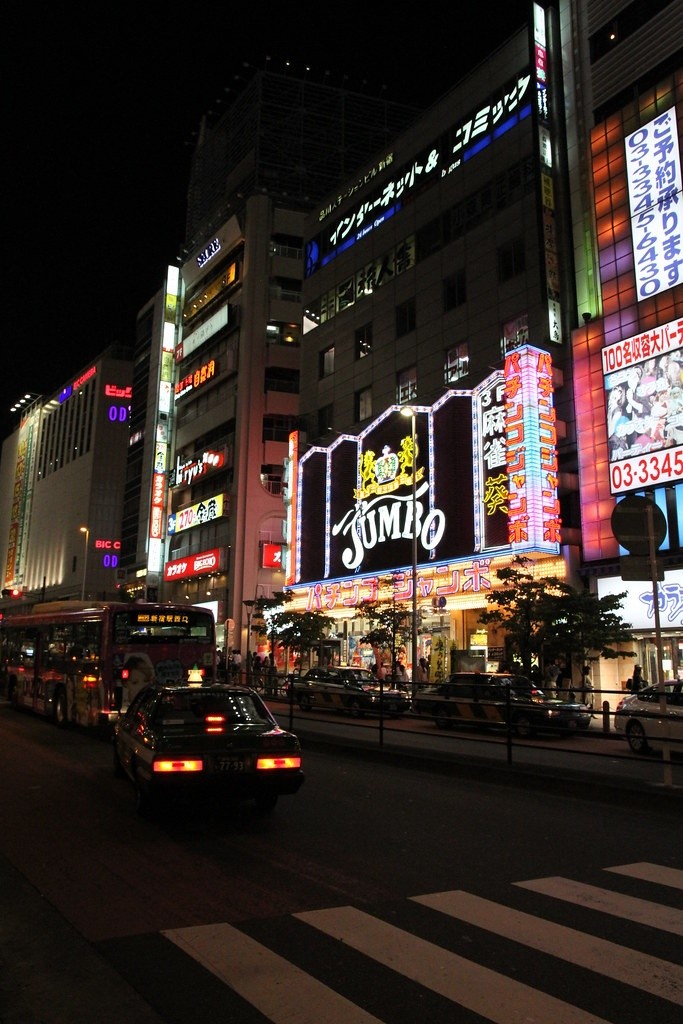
[606,349,682,460]
[503,659,648,720]
[120,653,157,712]
[214,643,431,692]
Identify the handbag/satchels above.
[626,679,633,689]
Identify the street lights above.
[399,404,419,699]
[79,526,90,602]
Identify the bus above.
[1,601,218,747]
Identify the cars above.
[290,665,411,719]
[412,670,591,740]
[613,680,683,757]
[115,669,306,816]
